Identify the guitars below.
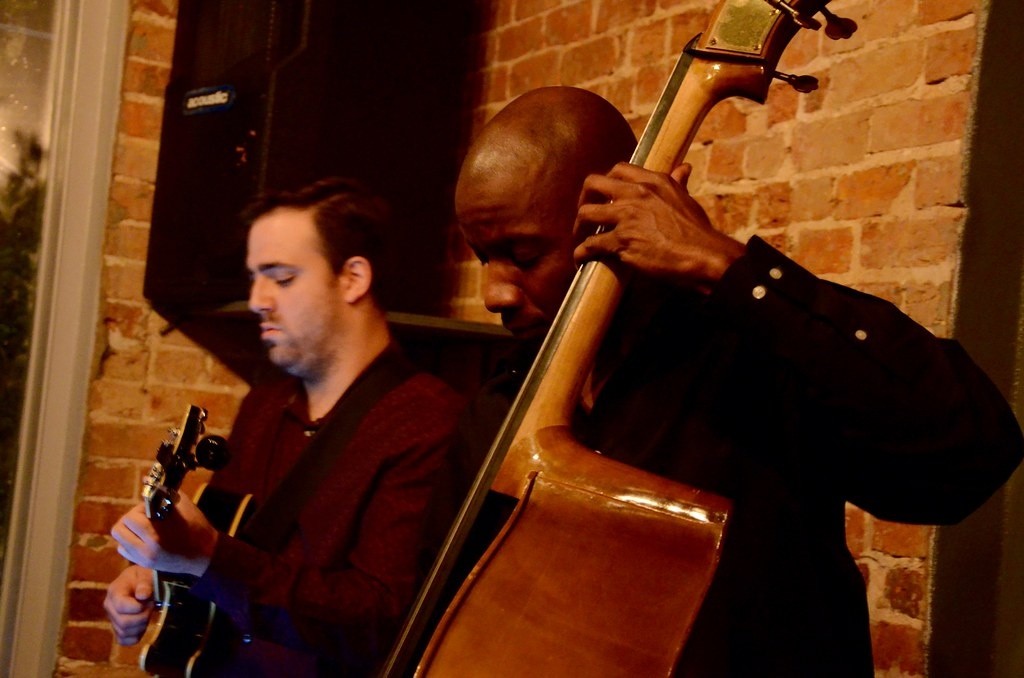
[139,403,255,678]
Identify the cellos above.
[376,0,861,678]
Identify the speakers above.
[144,0,495,305]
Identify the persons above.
[100,174,469,678]
[415,85,1022,678]
